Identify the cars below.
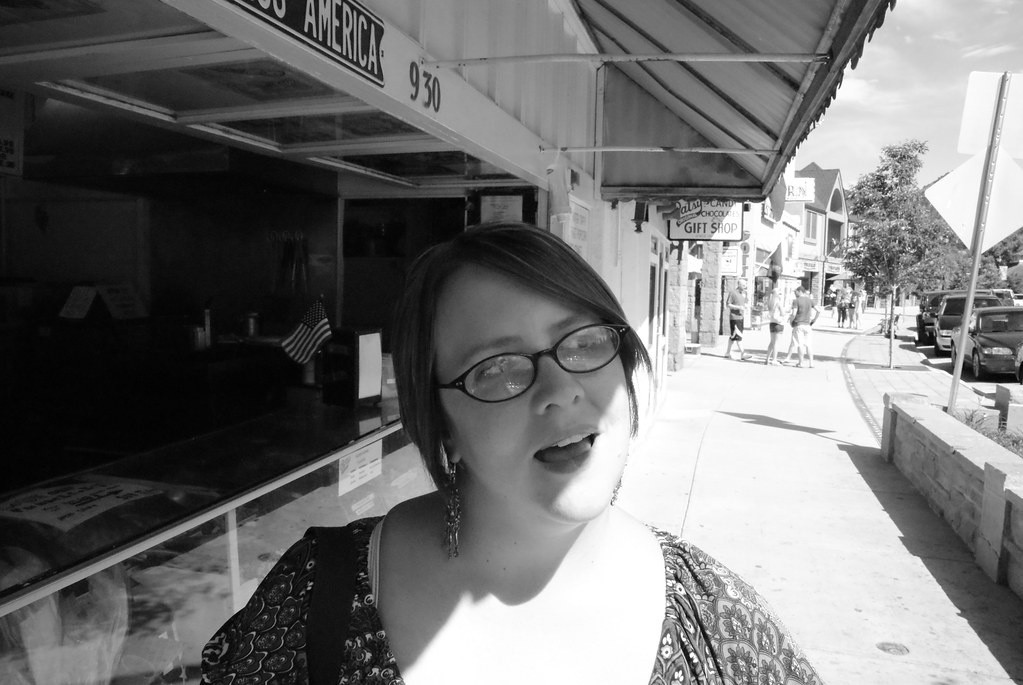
[917,287,1023,346]
[950,306,1023,384]
[929,294,1006,357]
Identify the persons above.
[780,286,821,368]
[200,220,821,685]
[765,288,785,366]
[724,279,753,360]
[836,283,867,330]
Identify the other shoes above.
[809,366,814,368]
[740,354,752,360]
[854,328,857,329]
[847,327,852,328]
[723,355,731,358]
[796,364,801,367]
[780,360,791,364]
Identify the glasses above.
[435,324,629,403]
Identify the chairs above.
[982,319,994,331]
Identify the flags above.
[281,296,332,365]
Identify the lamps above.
[828,244,841,257]
